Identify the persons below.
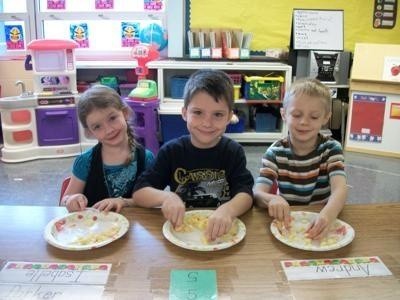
[59,84,156,215]
[134,68,255,243]
[251,76,347,239]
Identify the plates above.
[161,210,248,253]
[43,208,130,253]
[270,210,356,253]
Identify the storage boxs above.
[157,98,190,145]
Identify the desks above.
[0,201,399,300]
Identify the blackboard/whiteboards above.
[291,8,344,52]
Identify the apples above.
[8,263,108,270]
[391,66,400,76]
[285,258,377,267]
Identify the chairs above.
[59,177,69,207]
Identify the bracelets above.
[119,196,131,206]
[64,195,70,204]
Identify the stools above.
[125,98,162,154]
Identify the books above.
[211,32,216,48]
[199,31,204,47]
[244,32,252,47]
[227,32,231,48]
[187,31,196,46]
[236,31,242,47]
[222,31,226,49]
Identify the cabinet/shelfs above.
[156,59,292,147]
